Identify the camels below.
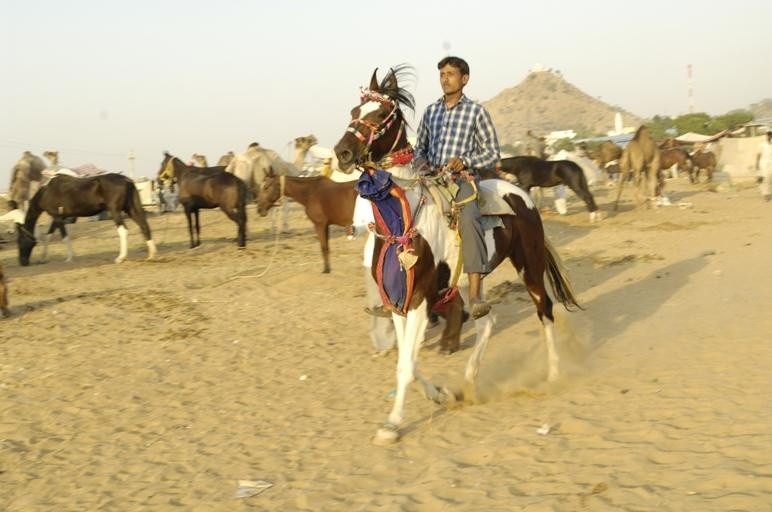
[225,135,318,235]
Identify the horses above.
[613,124,661,211]
[10,150,59,210]
[500,155,601,222]
[660,148,691,171]
[689,150,716,185]
[333,62,585,445]
[191,151,235,167]
[256,165,374,273]
[156,151,249,250]
[15,172,158,266]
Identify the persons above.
[363,56,500,320]
